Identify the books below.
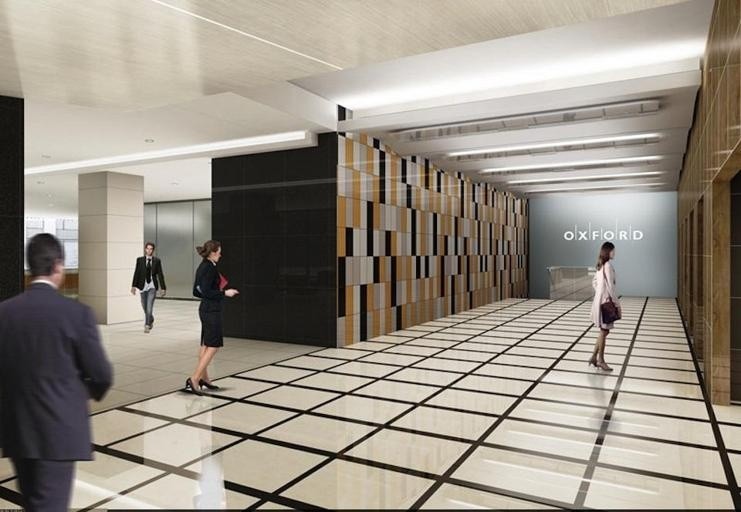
[195,271,229,294]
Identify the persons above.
[184,239,240,396]
[588,241,621,371]
[130,242,167,333]
[0,231,116,511]
